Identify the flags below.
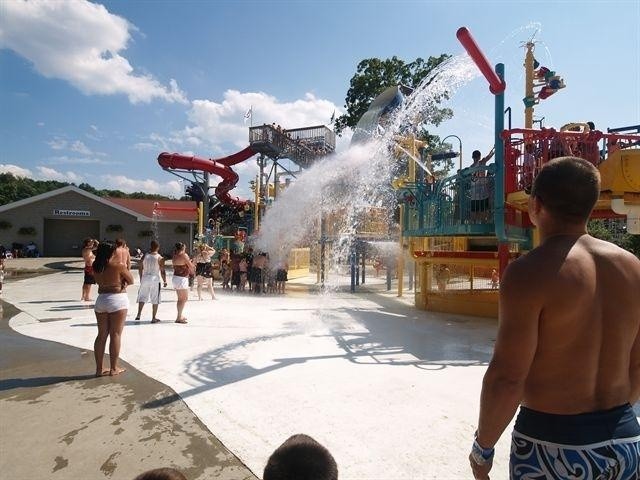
[243,106,252,123]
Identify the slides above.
[158,145,260,213]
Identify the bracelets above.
[471,428,497,461]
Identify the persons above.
[263,433,340,480]
[470,156,640,480]
[191,242,290,303]
[260,120,329,171]
[80,236,101,302]
[107,237,131,271]
[170,241,197,324]
[134,239,168,324]
[465,120,596,227]
[88,239,134,379]
